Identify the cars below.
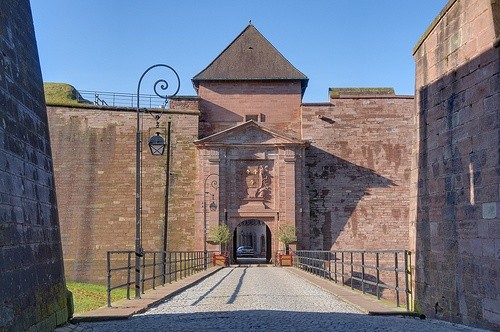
[236,246,255,258]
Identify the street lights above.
[133,63,181,298]
